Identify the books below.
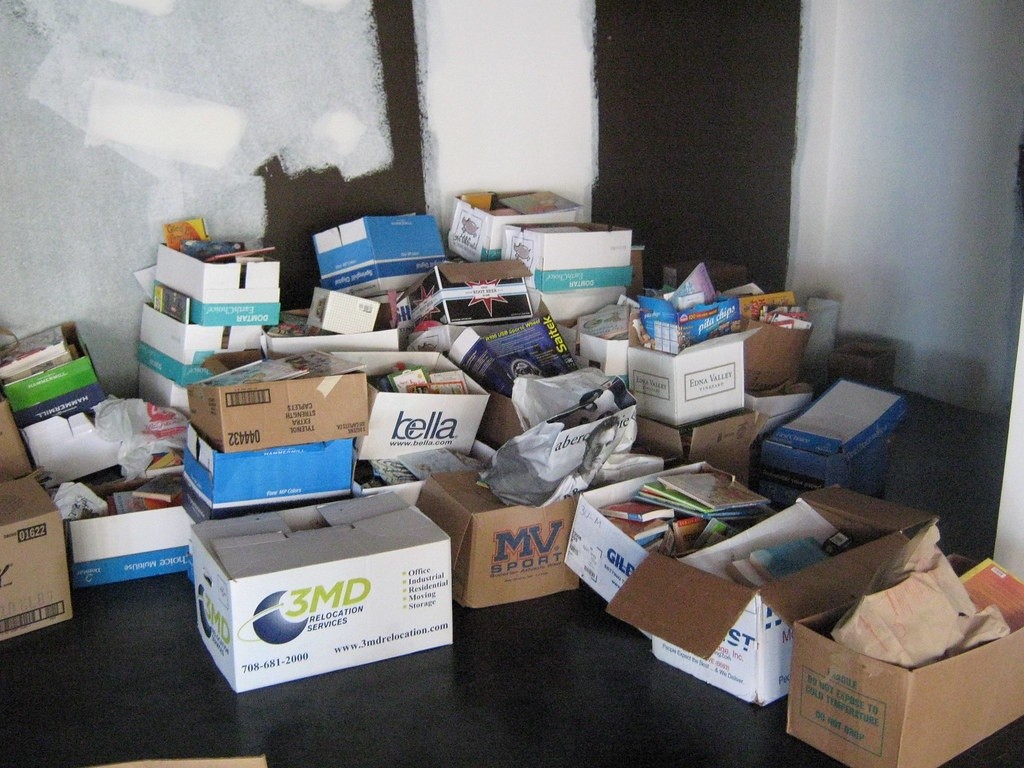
[0,190,1024,646]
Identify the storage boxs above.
[741,316,815,392]
[605,481,941,707]
[826,339,897,391]
[662,259,747,292]
[636,407,769,488]
[312,213,446,297]
[564,460,777,604]
[759,439,889,511]
[416,469,579,609]
[0,242,579,588]
[1,477,73,641]
[448,189,582,263]
[744,381,815,466]
[557,320,629,391]
[190,491,455,695]
[500,222,633,321]
[633,294,742,356]
[771,378,908,460]
[786,553,1024,768]
[628,310,762,428]
